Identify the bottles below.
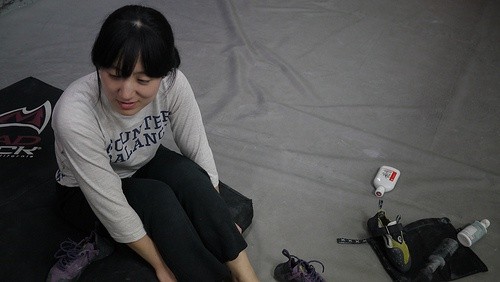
[457,219,490,246]
[427,238,458,273]
[374,166,401,197]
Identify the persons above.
[51,4,260,282]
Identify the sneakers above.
[368,210,413,273]
[273,249,329,282]
[47,230,100,282]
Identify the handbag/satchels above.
[338,209,488,282]
